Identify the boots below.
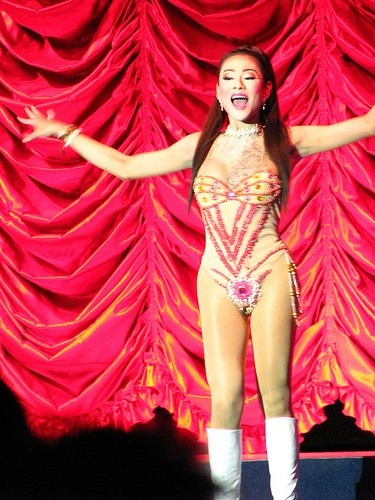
[264,417,300,500]
[206,428,243,500]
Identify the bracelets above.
[57,124,75,155]
[63,128,81,148]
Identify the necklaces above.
[223,124,264,137]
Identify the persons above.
[16,45,375,500]
